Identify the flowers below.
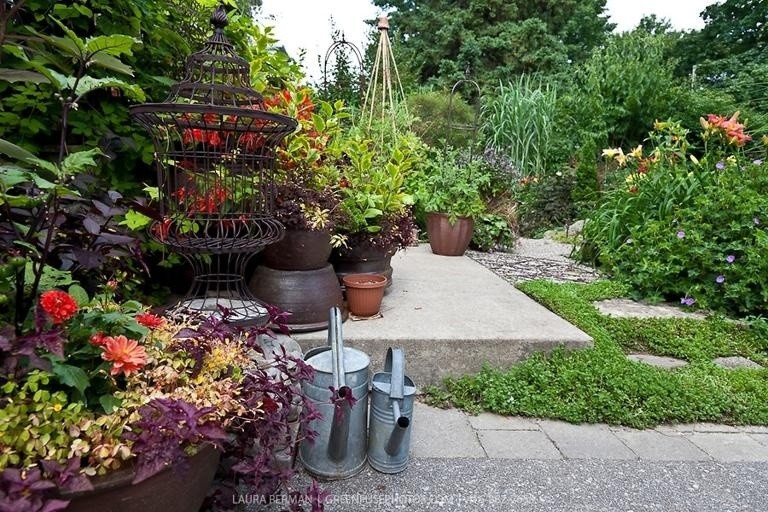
[0,285,330,512]
[173,83,423,252]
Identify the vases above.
[246,217,343,325]
[53,435,224,512]
[344,242,402,318]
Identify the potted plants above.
[417,156,501,258]
